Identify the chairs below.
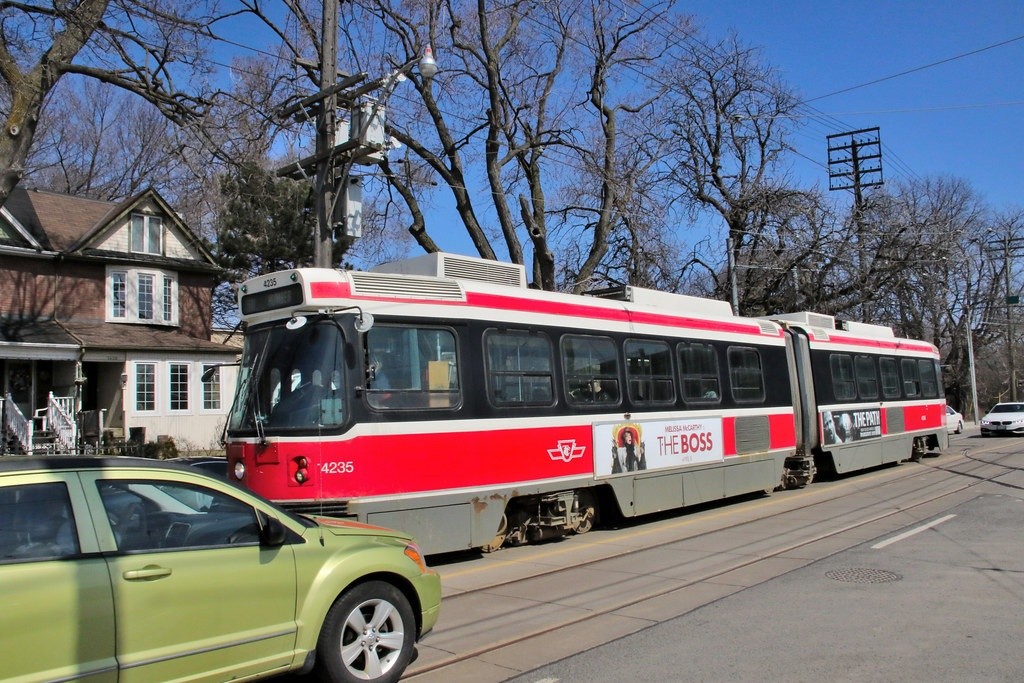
[11,487,64,558]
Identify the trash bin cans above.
[130,427,146,445]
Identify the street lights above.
[314,43,442,267]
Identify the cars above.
[151,455,228,510]
[946,405,964,435]
[0,453,442,683]
[980,402,1024,437]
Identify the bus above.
[199,250,950,558]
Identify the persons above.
[57,501,133,557]
[823,410,843,445]
[351,351,390,405]
